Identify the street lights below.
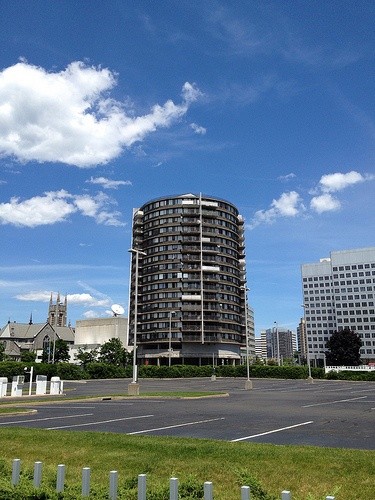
[274,321,280,367]
[302,303,314,384]
[240,284,252,390]
[128,247,147,384]
